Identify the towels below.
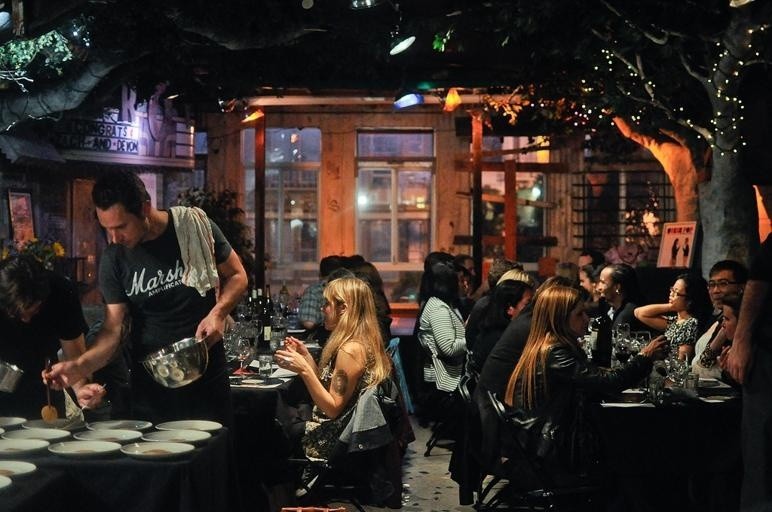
[168,206,221,297]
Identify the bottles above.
[251,290,256,314]
[280,281,289,311]
[248,297,253,309]
[258,308,272,354]
[258,289,265,311]
[265,284,273,313]
[597,298,612,370]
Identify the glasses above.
[707,279,735,290]
[669,286,688,298]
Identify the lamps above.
[393,76,421,109]
[385,0,416,57]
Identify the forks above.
[58,384,107,430]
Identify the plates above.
[1,429,71,442]
[170,368,185,382]
[156,365,169,377]
[154,419,223,433]
[170,361,177,368]
[0,461,37,477]
[0,417,27,427]
[47,441,122,458]
[20,418,89,430]
[87,420,152,431]
[119,442,195,460]
[140,430,211,442]
[73,430,143,443]
[0,439,50,456]
[0,476,13,491]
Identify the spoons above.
[41,358,57,424]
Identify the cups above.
[270,332,280,365]
[233,305,251,341]
[250,320,263,354]
[270,300,300,336]
[664,354,699,400]
[612,324,652,343]
[615,342,631,366]
[221,321,235,364]
[258,354,272,379]
[232,339,251,380]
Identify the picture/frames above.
[657,222,698,267]
[8,192,36,243]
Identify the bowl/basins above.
[0,360,25,394]
[139,336,209,389]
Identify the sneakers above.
[295,461,320,498]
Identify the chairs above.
[383,337,400,364]
[473,388,554,512]
[393,336,483,457]
[303,376,396,512]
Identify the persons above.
[690,293,745,390]
[578,263,599,291]
[599,263,636,334]
[413,252,453,341]
[275,276,395,497]
[42,170,249,512]
[454,265,469,321]
[298,255,350,330]
[693,260,748,366]
[14,196,30,225]
[0,250,107,421]
[474,276,616,456]
[351,261,393,350]
[418,263,469,392]
[507,284,670,511]
[467,266,531,363]
[478,259,522,299]
[351,254,365,268]
[634,275,705,362]
[727,145,772,512]
[455,256,478,279]
[475,281,536,381]
